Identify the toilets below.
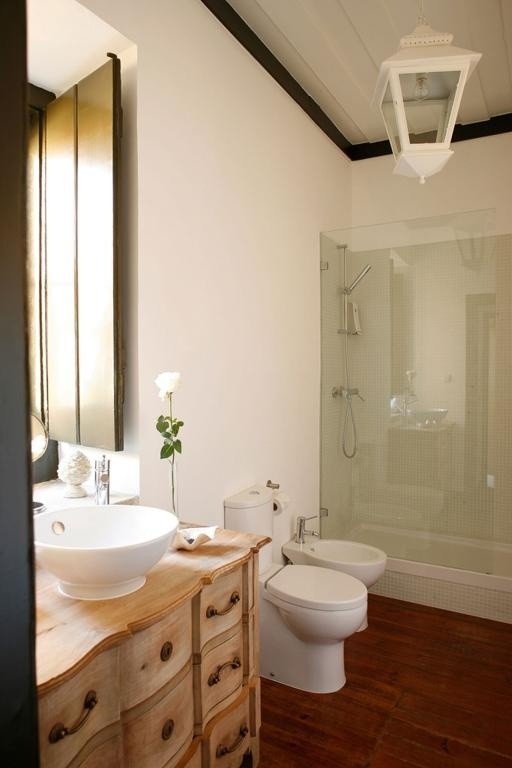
[348,442,444,550]
[222,483,369,696]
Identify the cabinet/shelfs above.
[387,423,455,497]
[34,521,276,767]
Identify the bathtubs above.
[343,524,512,627]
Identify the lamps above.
[449,214,503,268]
[364,1,485,191]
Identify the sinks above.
[408,408,448,425]
[282,534,387,633]
[33,505,179,584]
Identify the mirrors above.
[29,412,52,516]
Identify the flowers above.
[405,367,417,401]
[148,369,195,516]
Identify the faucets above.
[296,515,320,544]
[403,398,418,414]
[94,454,110,506]
